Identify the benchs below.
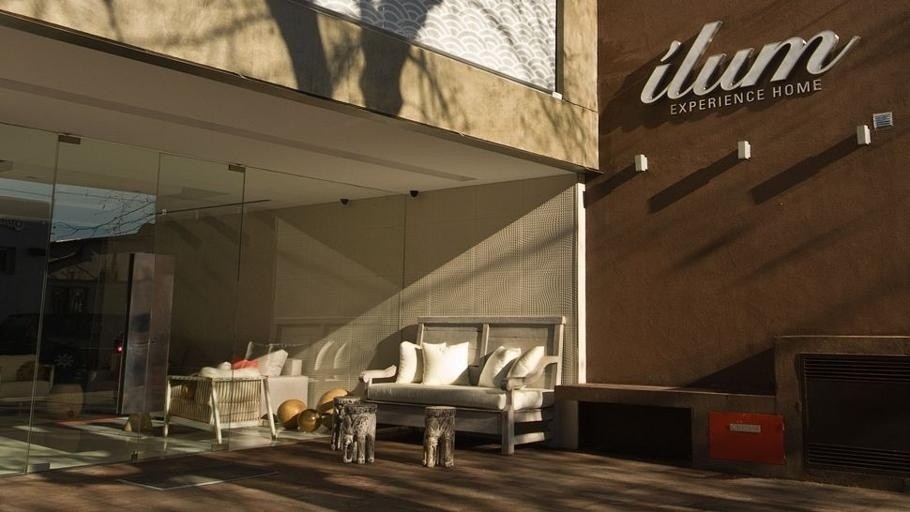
[557,380,777,472]
[357,315,567,456]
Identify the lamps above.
[634,124,871,174]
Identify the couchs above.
[0,355,50,413]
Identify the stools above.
[331,394,457,470]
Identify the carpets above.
[114,455,282,491]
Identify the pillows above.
[394,338,546,393]
[199,348,288,382]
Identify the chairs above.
[263,358,309,417]
[159,369,278,446]
